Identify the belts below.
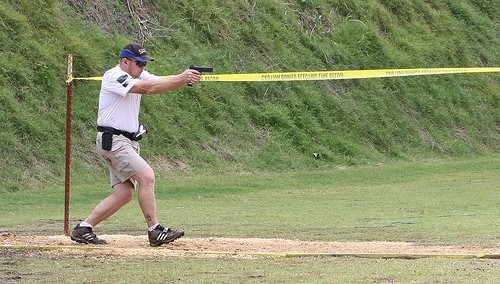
[97,126,139,142]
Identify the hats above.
[119,43,155,63]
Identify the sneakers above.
[70,223,108,245]
[147,224,185,247]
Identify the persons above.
[70,42,203,247]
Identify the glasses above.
[126,57,147,66]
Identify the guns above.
[187,65,213,86]
